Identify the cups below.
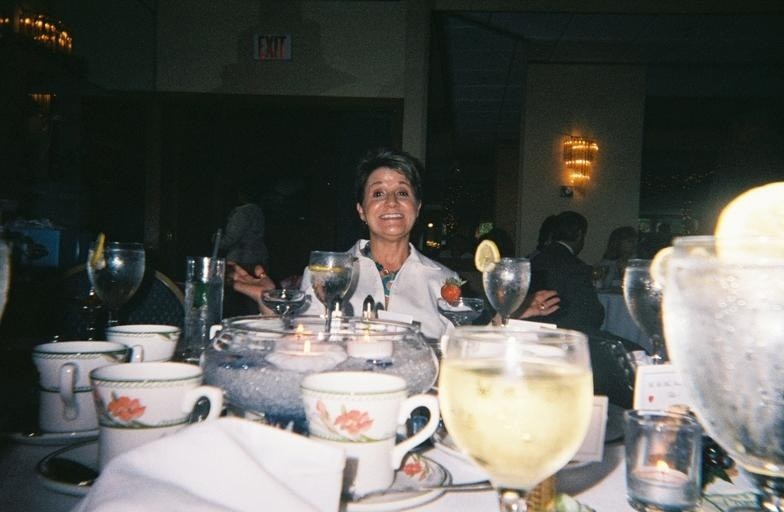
[88,360,223,476]
[261,290,307,331]
[183,256,227,364]
[104,324,182,363]
[623,410,703,512]
[30,341,129,432]
[438,297,484,329]
[86,241,146,326]
[309,250,354,339]
[299,371,439,498]
[439,329,594,512]
[623,259,666,366]
[482,256,532,327]
[660,235,783,512]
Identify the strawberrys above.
[440,277,467,307]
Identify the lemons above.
[90,232,106,267]
[474,239,501,273]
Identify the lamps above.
[2,0,73,60]
[561,136,601,203]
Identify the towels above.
[70,418,347,512]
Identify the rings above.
[538,302,546,312]
[257,270,266,277]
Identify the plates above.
[605,404,630,442]
[341,457,452,512]
[11,430,100,446]
[33,439,99,496]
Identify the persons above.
[521,213,559,263]
[593,226,639,287]
[219,183,269,316]
[225,151,561,320]
[530,210,635,411]
